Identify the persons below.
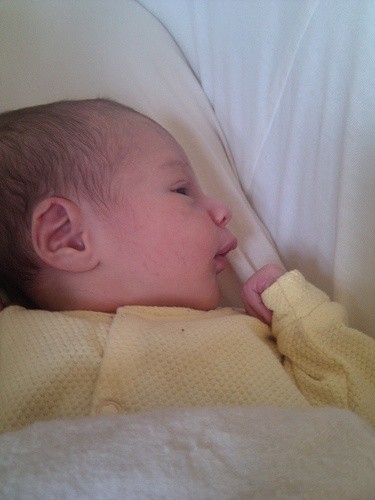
[1,100,375,436]
